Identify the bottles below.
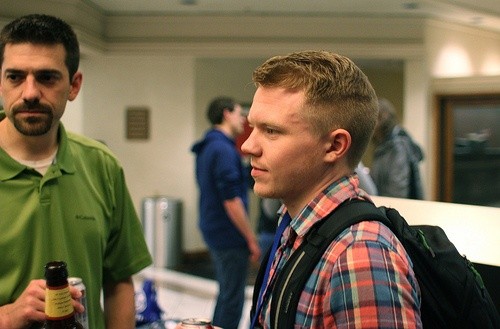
[43,262,82,329]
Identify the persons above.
[176,50,424,329]
[369,97,423,201]
[0,14,153,329]
[192,96,260,329]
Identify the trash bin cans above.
[143,196,181,268]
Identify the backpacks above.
[249,198,499,329]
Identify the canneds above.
[67,277,89,329]
